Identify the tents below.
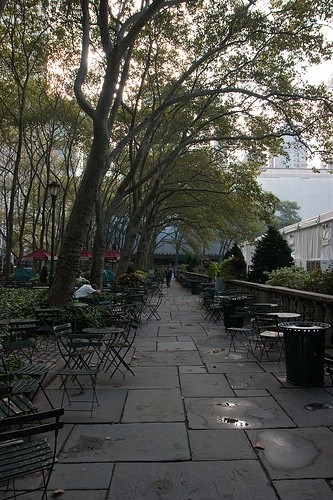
[104,249,119,271]
[79,251,92,266]
[22,248,58,267]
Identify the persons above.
[74,284,102,305]
[165,265,172,287]
[39,265,49,286]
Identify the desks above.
[268,311,301,321]
[219,295,245,301]
[254,302,279,308]
[83,327,124,334]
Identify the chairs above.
[197,282,333,389]
[0,278,165,500]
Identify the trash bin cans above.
[278,321,331,385]
[223,296,246,330]
[190,280,201,294]
